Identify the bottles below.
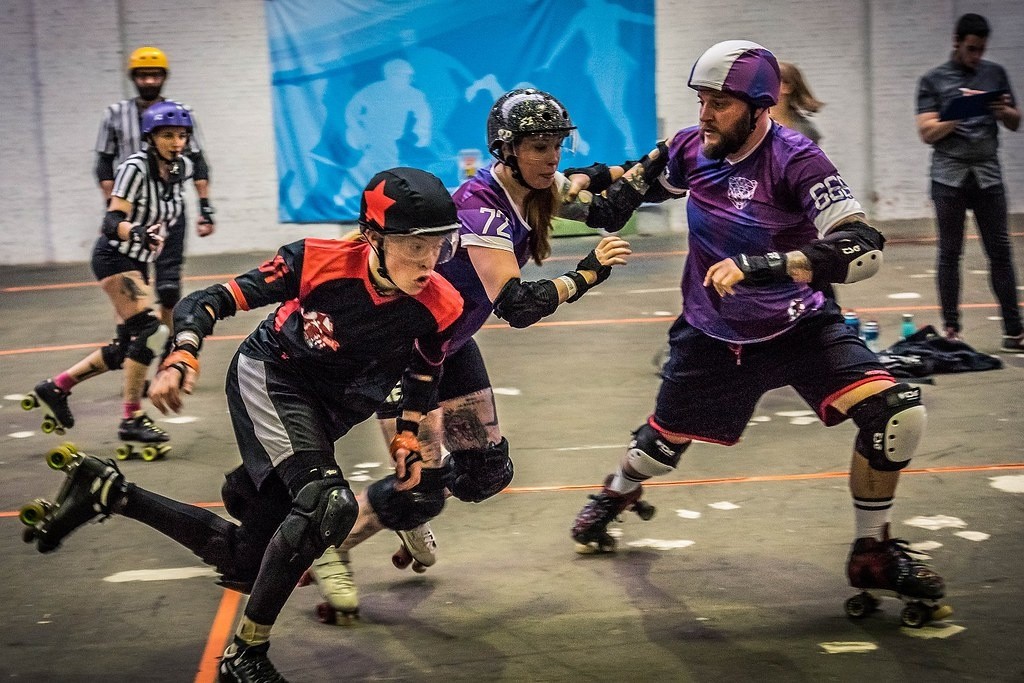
[899,313,917,340]
[862,321,881,354]
[844,310,861,336]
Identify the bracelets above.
[395,418,420,436]
[556,275,576,299]
[172,343,198,359]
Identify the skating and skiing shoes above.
[392,521,438,573]
[215,637,288,683]
[298,544,359,623]
[845,523,953,627]
[114,411,173,461]
[19,443,124,555]
[20,378,75,435]
[571,474,655,555]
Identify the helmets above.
[141,102,193,138]
[485,88,577,151]
[359,167,463,236]
[687,39,782,107]
[127,46,169,72]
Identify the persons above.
[90,45,216,399]
[560,38,953,628]
[296,88,633,627]
[769,60,828,145]
[15,167,463,683]
[913,12,1024,355]
[20,102,195,462]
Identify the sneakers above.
[943,328,1024,352]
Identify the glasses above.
[136,72,165,78]
[509,140,565,160]
[151,132,191,138]
[383,234,454,265]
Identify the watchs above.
[564,270,589,303]
